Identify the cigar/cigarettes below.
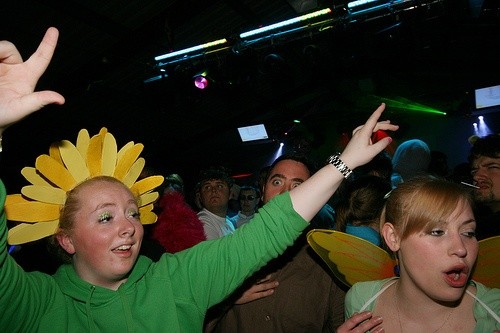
[460,182,480,189]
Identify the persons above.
[138,141,500,250]
[204,155,346,333]
[0,26,400,333]
[338,175,500,333]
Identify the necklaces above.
[399,309,451,333]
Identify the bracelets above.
[330,152,353,179]
